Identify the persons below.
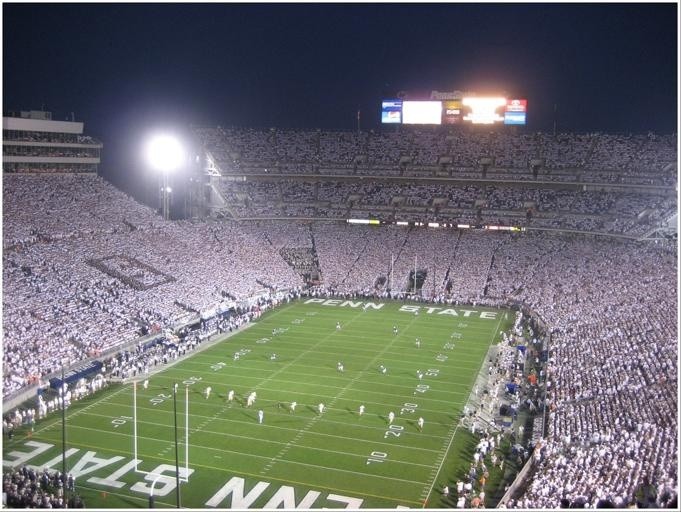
[2,126,677,507]
